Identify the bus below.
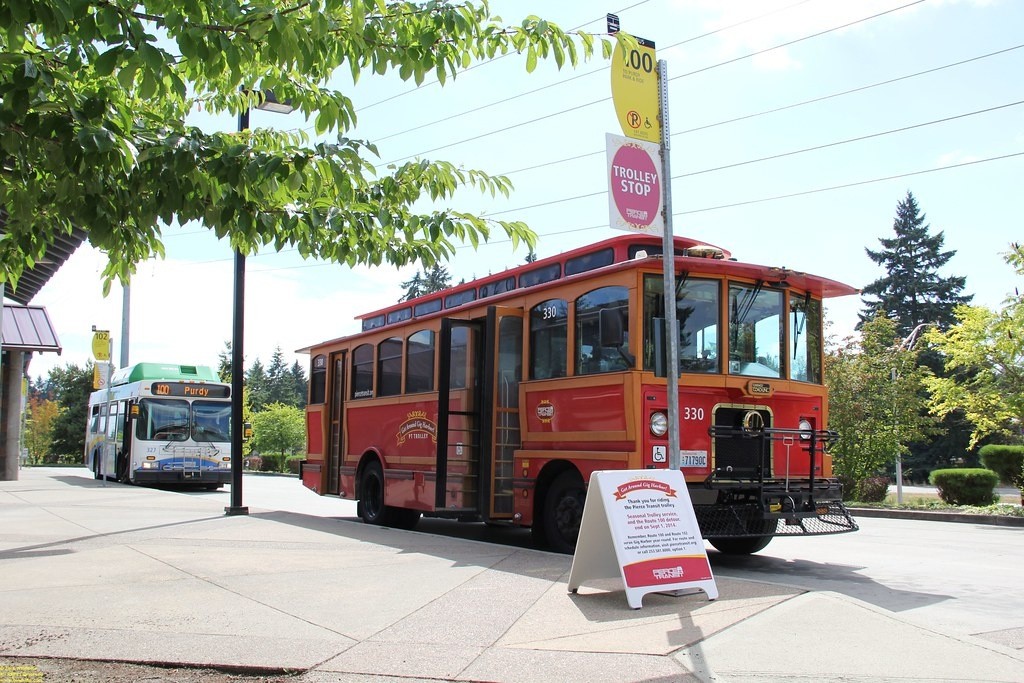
[85,362,253,491]
[295,233,861,539]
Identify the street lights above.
[226,82,294,506]
[890,323,940,506]
